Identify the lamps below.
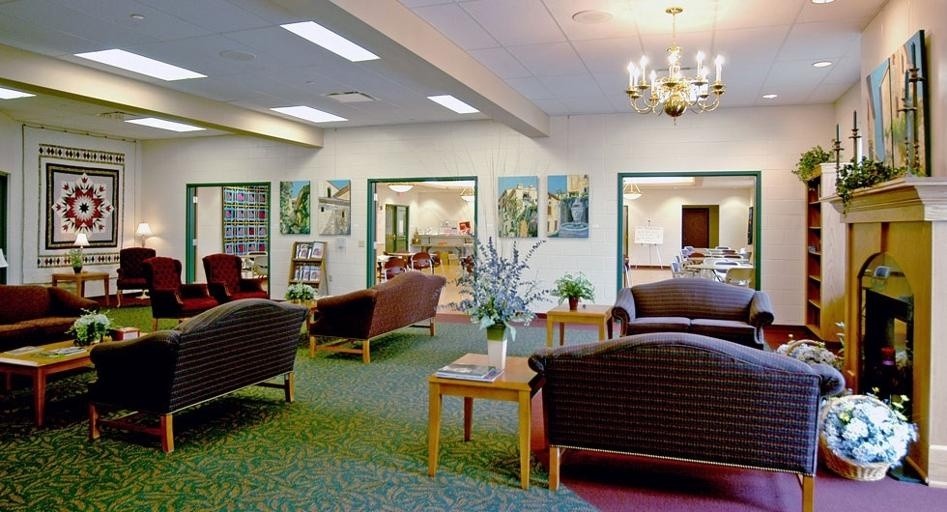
[73,234,90,257]
[625,8,726,125]
[623,184,642,199]
[0,248,9,267]
[460,188,475,201]
[136,219,150,247]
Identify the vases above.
[487,323,507,371]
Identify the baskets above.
[817,393,899,481]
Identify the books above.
[294,242,324,282]
[433,363,506,382]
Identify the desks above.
[427,352,542,490]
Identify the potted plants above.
[69,249,83,272]
[285,283,315,305]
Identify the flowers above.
[432,231,557,342]
[821,387,920,468]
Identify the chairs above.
[670,246,754,288]
[116,247,267,332]
[384,250,441,280]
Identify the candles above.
[854,110,857,128]
[910,42,916,66]
[836,124,839,142]
[906,70,909,98]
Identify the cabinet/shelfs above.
[804,162,853,341]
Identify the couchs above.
[309,270,446,363]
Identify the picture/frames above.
[748,207,753,245]
